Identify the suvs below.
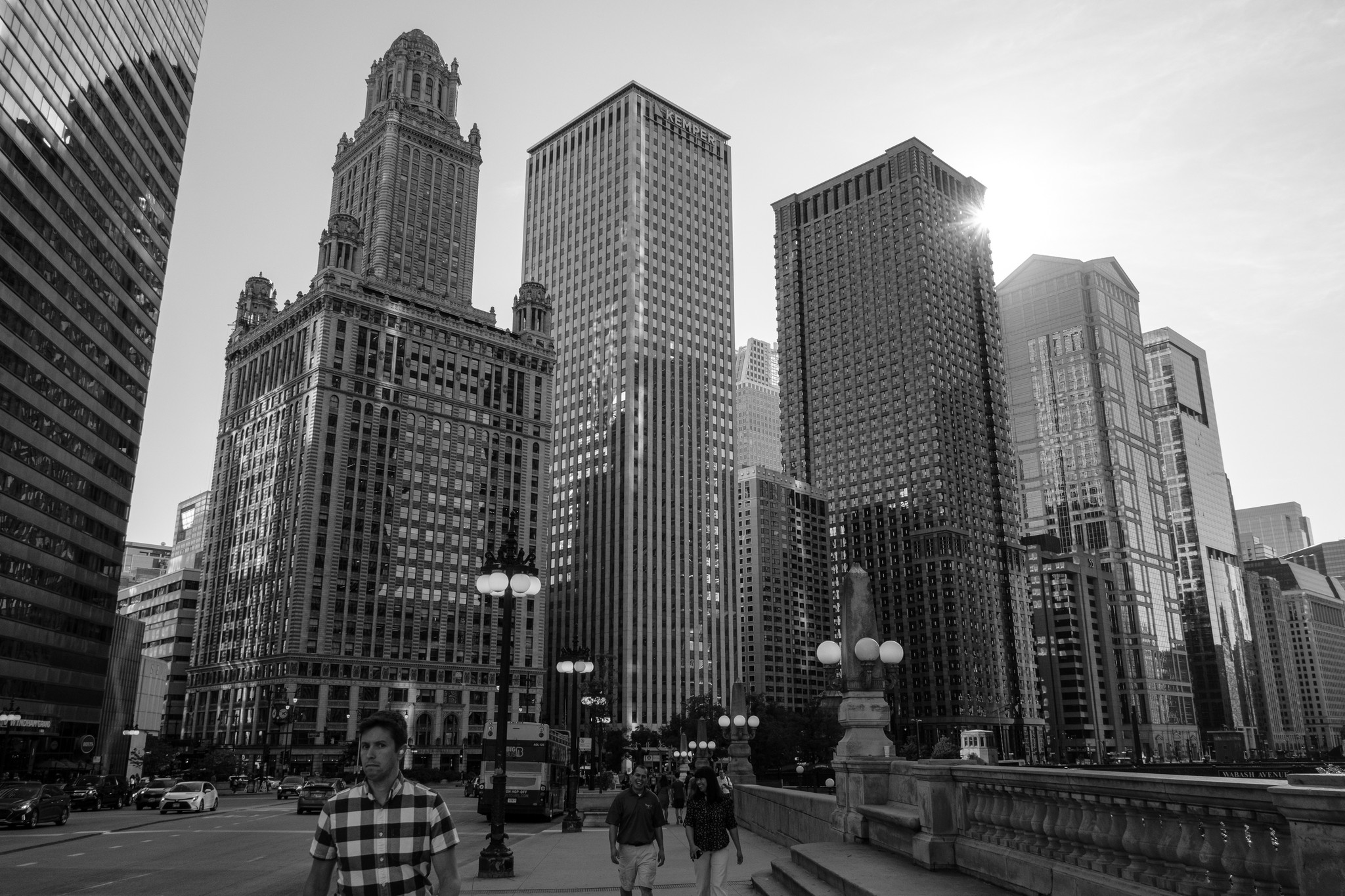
[63,774,133,812]
[135,777,185,810]
[277,776,309,800]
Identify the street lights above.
[581,688,611,790]
[474,511,541,879]
[555,635,594,832]
[673,732,694,780]
[688,716,716,766]
[825,778,835,795]
[796,765,804,790]
[819,560,904,757]
[718,677,760,784]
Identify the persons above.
[605,765,666,896]
[303,709,461,896]
[129,773,140,789]
[683,766,743,896]
[265,778,270,793]
[231,779,238,794]
[621,771,700,825]
[0,771,20,781]
[716,768,733,800]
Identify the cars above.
[297,781,337,815]
[255,775,281,789]
[323,778,346,791]
[0,783,71,830]
[464,776,480,798]
[159,781,219,815]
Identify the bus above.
[478,720,572,822]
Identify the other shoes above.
[679,816,683,824]
[666,820,669,824]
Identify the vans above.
[229,775,249,787]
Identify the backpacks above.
[651,778,656,785]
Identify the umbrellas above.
[38,759,93,769]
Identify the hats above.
[652,773,656,776]
